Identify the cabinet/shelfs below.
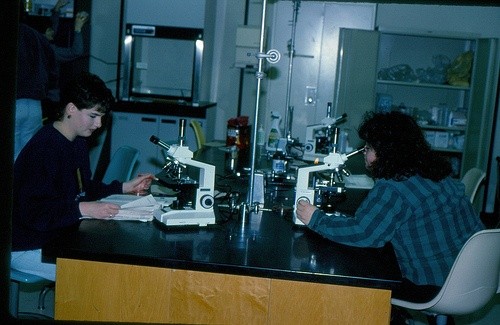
[333,27,500,211]
[103,96,217,187]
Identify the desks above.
[42,139,403,325]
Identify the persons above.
[9,70,154,282]
[296,111,488,325]
[1,0,89,102]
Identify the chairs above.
[461,167,487,207]
[391,229,500,325]
[8,268,57,319]
[101,144,139,186]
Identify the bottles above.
[272,148,287,173]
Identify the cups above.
[225,124,252,158]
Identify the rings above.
[109,213,112,218]
